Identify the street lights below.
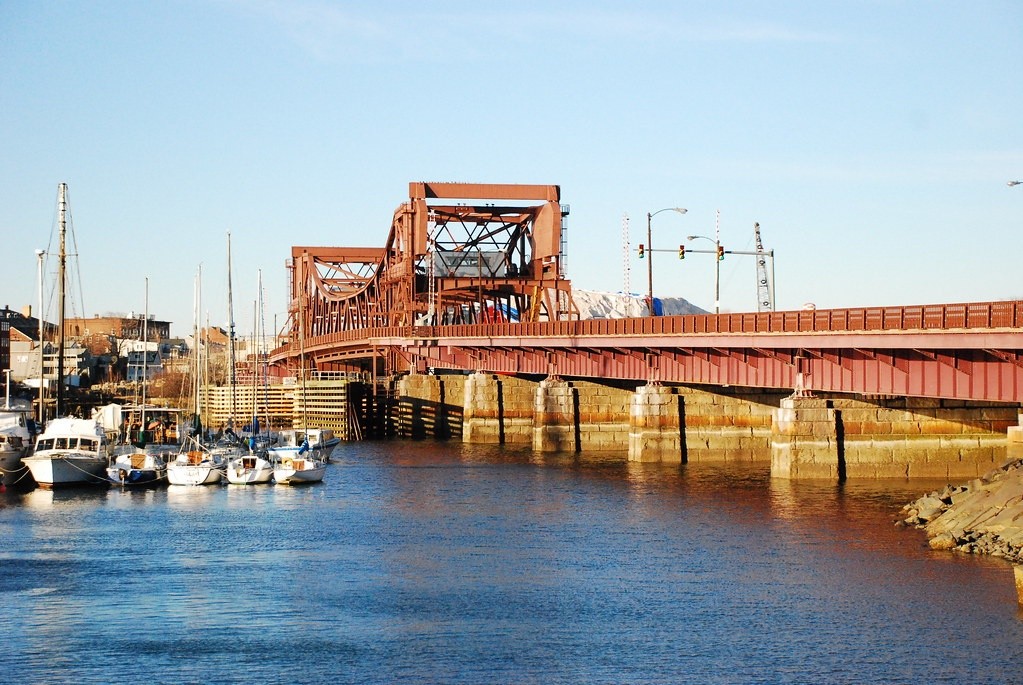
[646,208,688,316]
[687,234,721,314]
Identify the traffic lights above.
[639,244,644,258]
[718,246,725,260]
[679,245,685,259]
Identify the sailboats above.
[0,181,341,491]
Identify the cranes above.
[754,221,772,311]
[622,210,631,318]
